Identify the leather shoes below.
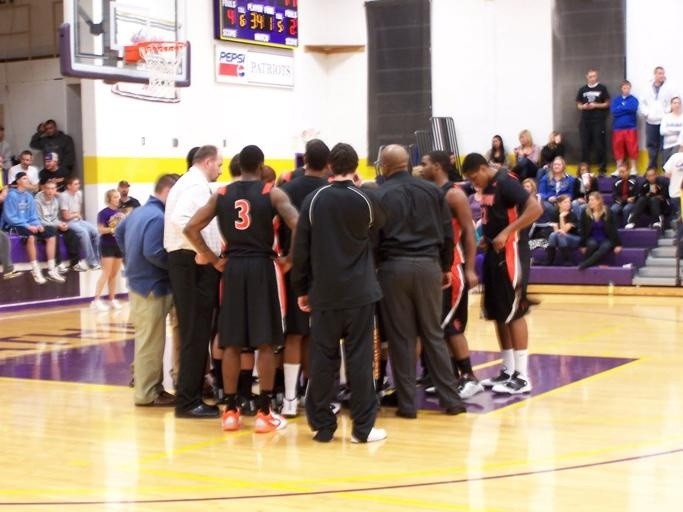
[446,406,467,414]
[135,395,174,406]
[394,409,417,418]
[161,389,176,398]
[175,403,218,419]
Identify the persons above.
[446,151,463,182]
[626,167,672,228]
[546,196,581,266]
[664,143,683,218]
[571,162,598,201]
[32,181,88,273]
[182,145,299,434]
[538,156,574,238]
[0,155,8,227]
[538,132,565,181]
[59,176,104,269]
[521,178,539,240]
[179,148,200,179]
[261,166,277,187]
[580,192,623,271]
[510,129,539,183]
[660,98,683,174]
[484,135,510,170]
[467,180,484,236]
[610,80,639,176]
[461,152,543,395]
[575,67,609,174]
[164,145,226,418]
[293,143,388,443]
[610,165,639,229]
[0,126,11,168]
[375,143,466,420]
[210,152,256,403]
[116,180,141,277]
[38,151,71,193]
[277,145,304,185]
[28,120,74,174]
[89,188,123,311]
[114,176,176,406]
[1,172,66,285]
[640,66,674,170]
[8,151,40,193]
[419,151,484,399]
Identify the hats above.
[119,180,130,188]
[10,172,27,185]
[44,152,58,161]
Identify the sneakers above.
[91,264,100,268]
[350,427,386,442]
[624,223,634,229]
[221,406,240,430]
[241,399,255,415]
[73,263,87,272]
[629,167,637,176]
[2,270,22,278]
[90,300,107,312]
[491,370,531,395]
[329,402,341,415]
[455,377,484,399]
[280,397,297,416]
[30,271,46,285]
[610,169,620,177]
[57,264,66,273]
[253,410,287,433]
[652,222,662,229]
[47,270,65,283]
[480,368,513,388]
[215,389,224,404]
[109,300,121,309]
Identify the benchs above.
[617,226,659,246]
[528,265,635,286]
[0,228,87,270]
[608,210,674,227]
[597,175,670,193]
[534,246,647,268]
[604,195,681,209]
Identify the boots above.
[562,245,576,267]
[540,246,556,265]
[583,248,594,260]
[575,251,600,271]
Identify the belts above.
[388,255,443,265]
